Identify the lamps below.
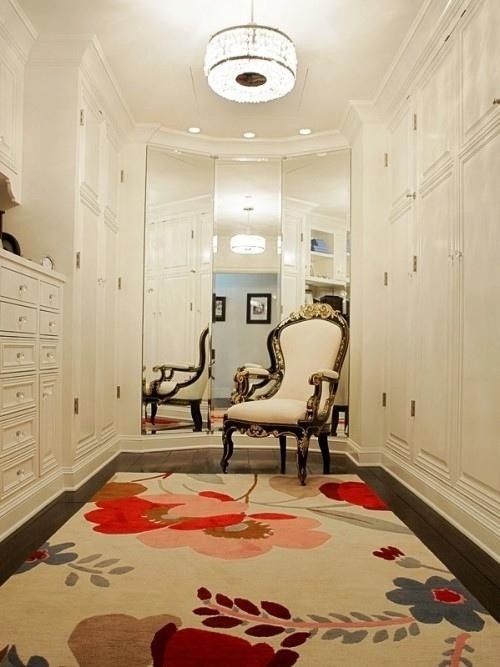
[229,208,266,255]
[203,0,297,103]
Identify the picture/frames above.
[247,294,272,324]
[213,297,226,321]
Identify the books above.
[311,237,331,253]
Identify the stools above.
[331,405,349,436]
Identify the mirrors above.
[214,158,282,433]
[276,149,353,438]
[141,144,214,435]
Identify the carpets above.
[142,415,209,434]
[0,473,500,667]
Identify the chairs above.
[218,302,349,486]
[143,323,210,435]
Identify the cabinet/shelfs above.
[0,0,38,209]
[377,0,499,563]
[282,209,304,316]
[304,205,350,306]
[0,249,66,542]
[73,38,136,492]
[144,195,213,414]
